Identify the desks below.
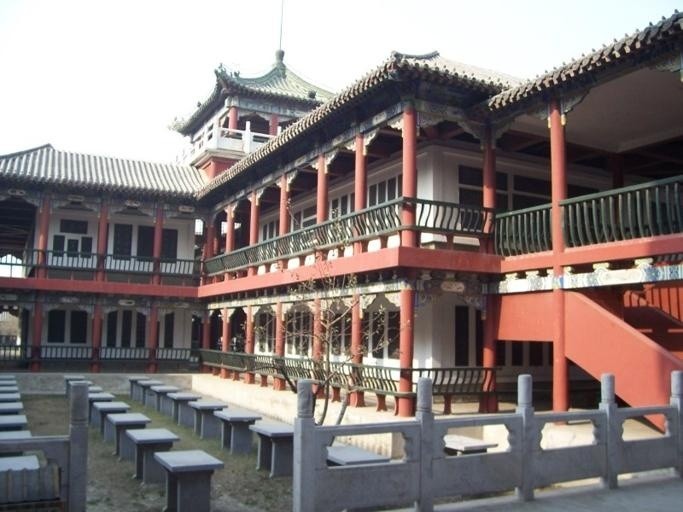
[106,412,152,459]
[125,426,180,483]
[166,392,202,425]
[186,400,228,434]
[248,423,298,479]
[1,371,40,507]
[151,450,225,512]
[149,385,179,413]
[444,432,497,456]
[64,375,116,422]
[92,401,132,433]
[214,408,262,453]
[137,380,164,406]
[127,376,151,400]
[326,443,390,469]
